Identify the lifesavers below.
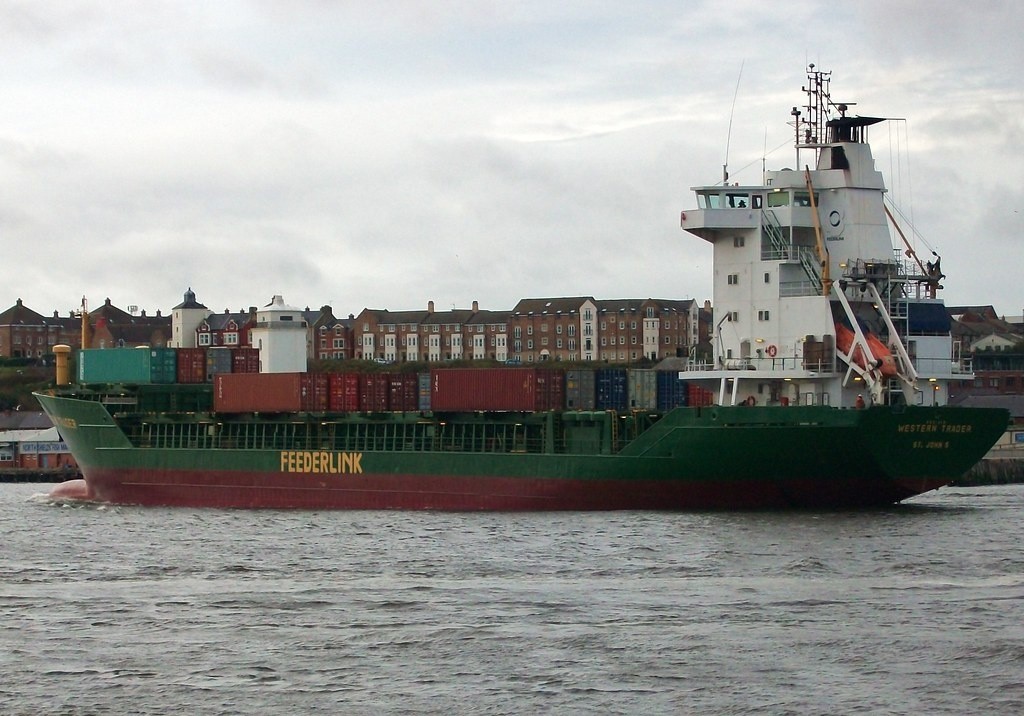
[768,345,777,357]
[746,395,756,406]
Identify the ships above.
[31,61,1012,513]
